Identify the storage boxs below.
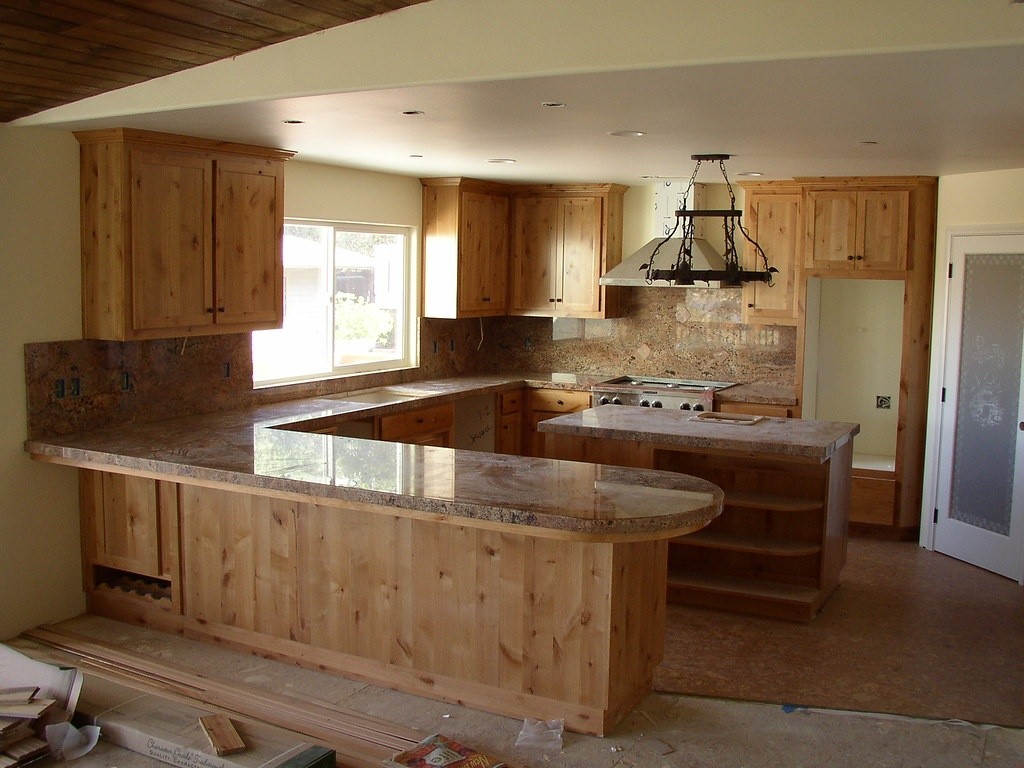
[35,655,337,768]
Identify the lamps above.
[638,154,780,288]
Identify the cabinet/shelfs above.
[736,180,802,327]
[804,174,942,272]
[714,401,795,419]
[508,183,630,320]
[380,387,591,461]
[848,475,897,527]
[419,176,512,320]
[72,127,298,342]
[545,433,854,623]
